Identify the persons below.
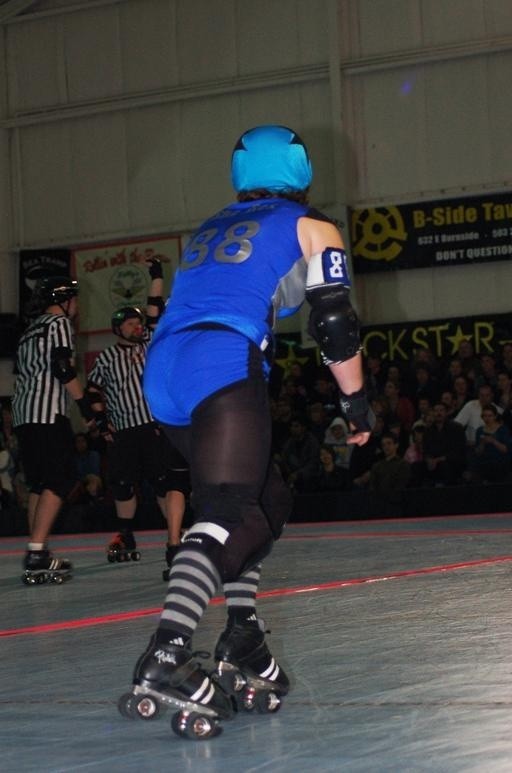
[79,252,186,563]
[3,337,511,522]
[133,121,381,725]
[11,274,99,578]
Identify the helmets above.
[111,306,145,336]
[41,277,78,305]
[230,126,313,193]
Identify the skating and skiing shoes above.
[162,542,181,581]
[118,630,237,739]
[211,614,289,714]
[21,550,72,583]
[106,533,142,562]
